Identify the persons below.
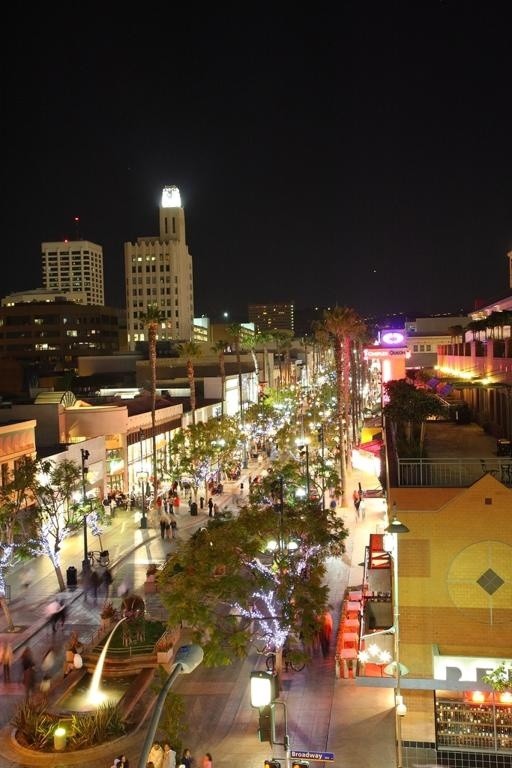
[109,740,213,768]
[157,477,181,541]
[188,496,193,514]
[300,607,333,660]
[209,497,214,518]
[352,491,367,520]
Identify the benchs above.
[144,555,174,592]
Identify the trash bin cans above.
[67,567,77,588]
[191,503,197,515]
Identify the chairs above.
[479,458,497,478]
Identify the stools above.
[496,438,512,456]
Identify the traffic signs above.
[290,751,335,762]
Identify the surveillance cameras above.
[396,703,406,718]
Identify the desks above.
[499,459,512,487]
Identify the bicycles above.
[262,645,306,674]
[87,548,111,569]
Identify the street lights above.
[266,537,301,671]
[395,697,408,768]
[208,376,369,544]
[138,476,150,529]
[78,447,95,575]
[139,645,207,768]
[248,663,312,768]
